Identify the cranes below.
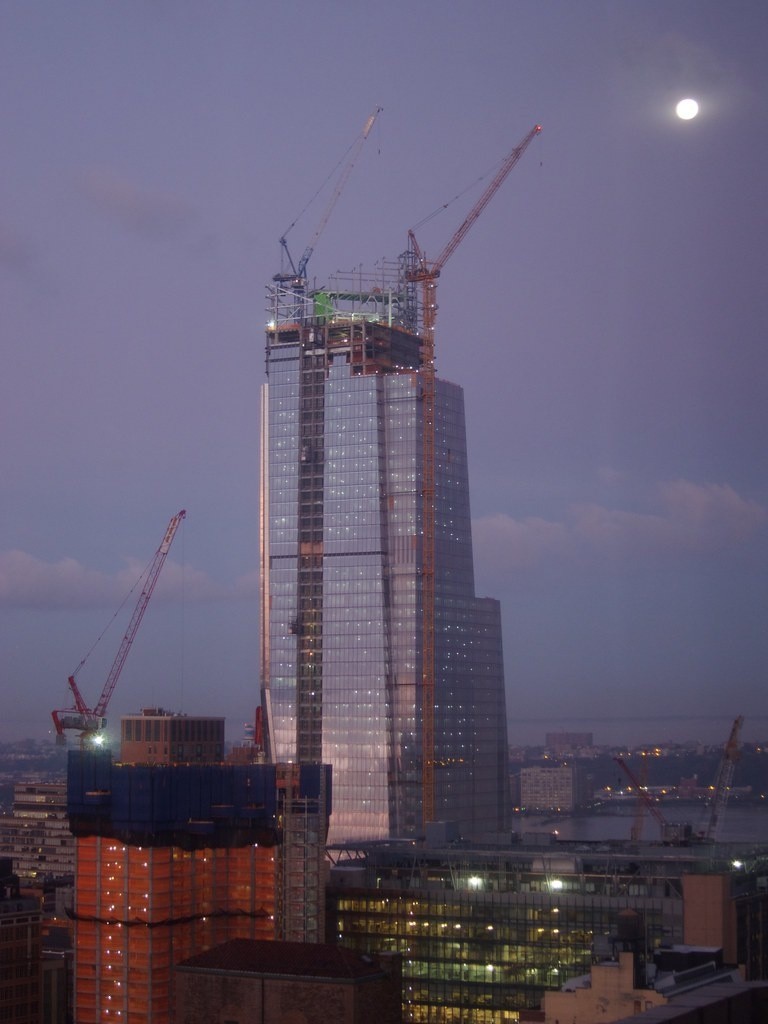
[50,508,186,753]
[396,124,545,836]
[271,104,385,323]
[616,712,746,862]
